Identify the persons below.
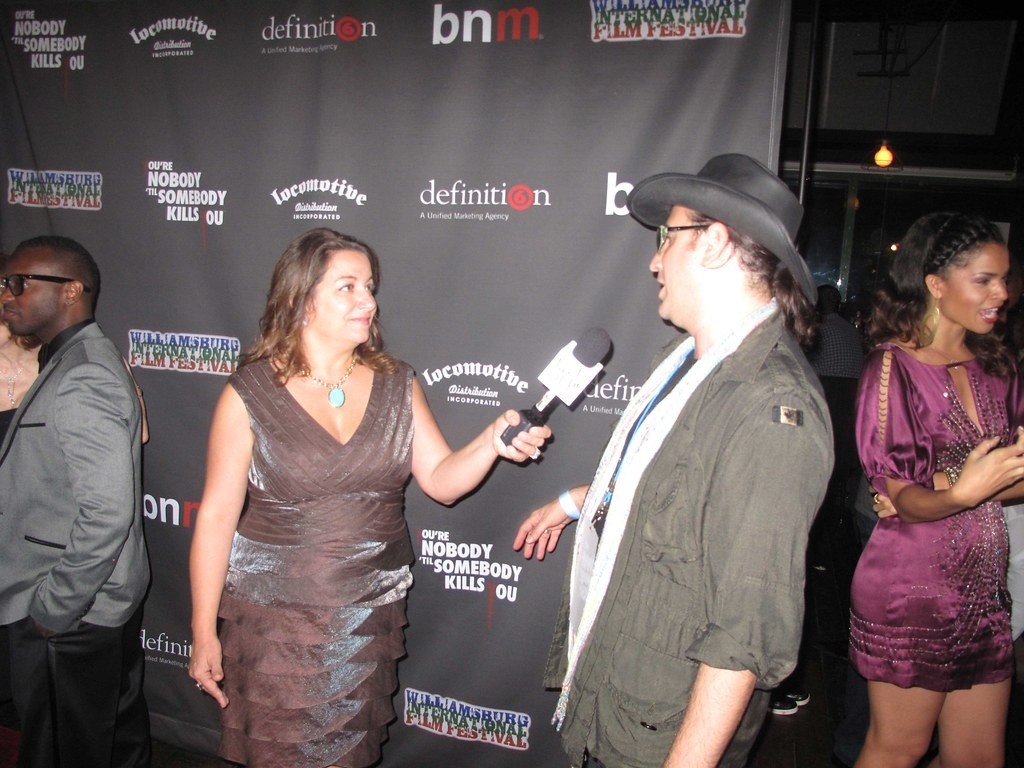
[512,154,834,768]
[847,209,1024,767]
[1,236,151,767]
[815,285,861,379]
[191,230,552,768]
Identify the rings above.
[530,448,541,459]
[195,683,201,690]
[544,530,550,533]
[874,494,879,503]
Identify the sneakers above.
[768,689,798,715]
[779,676,810,706]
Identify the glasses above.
[658,223,742,253]
[0,274,92,297]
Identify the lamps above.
[860,26,905,175]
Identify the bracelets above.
[558,492,581,521]
[943,467,958,486]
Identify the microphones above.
[500,326,613,446]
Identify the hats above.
[626,153,819,309]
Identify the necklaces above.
[300,360,356,408]
[950,358,960,369]
[0,367,24,405]
